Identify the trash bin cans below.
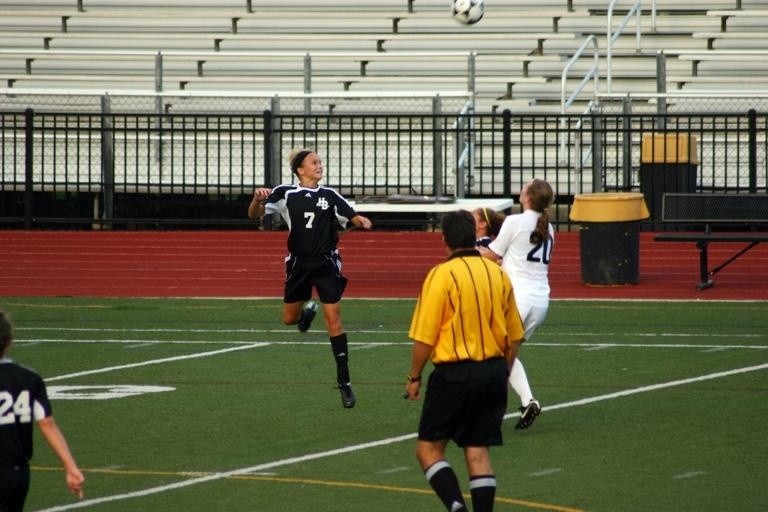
[575,135,699,285]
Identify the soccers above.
[452,0,484,24]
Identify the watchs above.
[407,372,421,385]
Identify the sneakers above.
[515,399,541,429]
[298,302,317,331]
[335,382,355,408]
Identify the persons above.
[1,311,86,512]
[404,209,525,512]
[467,207,508,267]
[246,148,373,409]
[472,178,558,430]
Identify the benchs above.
[653,193,768,291]
[0,0,768,198]
